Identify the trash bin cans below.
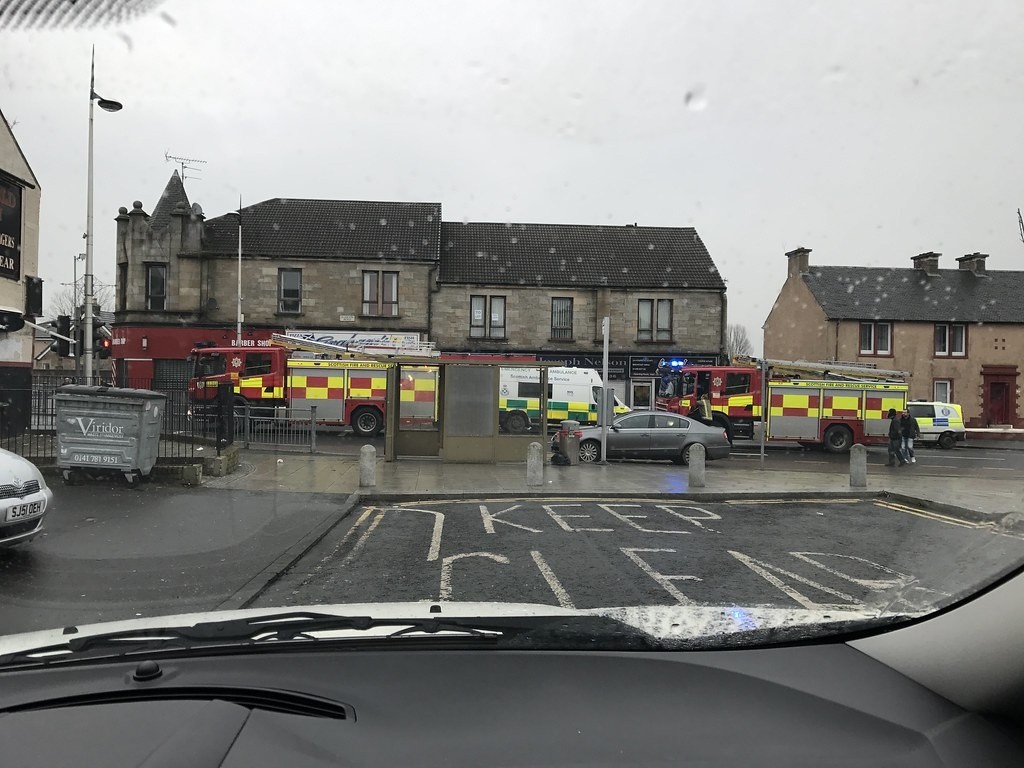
[53,384,167,487]
[559,420,580,465]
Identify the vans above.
[498,366,634,435]
[905,398,967,449]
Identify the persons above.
[696,394,713,426]
[900,409,920,463]
[885,409,906,467]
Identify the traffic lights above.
[51,315,70,356]
[92,317,106,357]
[98,337,111,360]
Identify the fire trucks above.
[186,325,538,439]
[655,354,914,455]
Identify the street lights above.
[72,252,88,306]
[81,41,123,392]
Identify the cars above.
[0,446,53,549]
[546,409,731,464]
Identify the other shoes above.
[911,457,916,462]
[885,462,895,466]
[905,459,910,464]
[898,462,906,467]
